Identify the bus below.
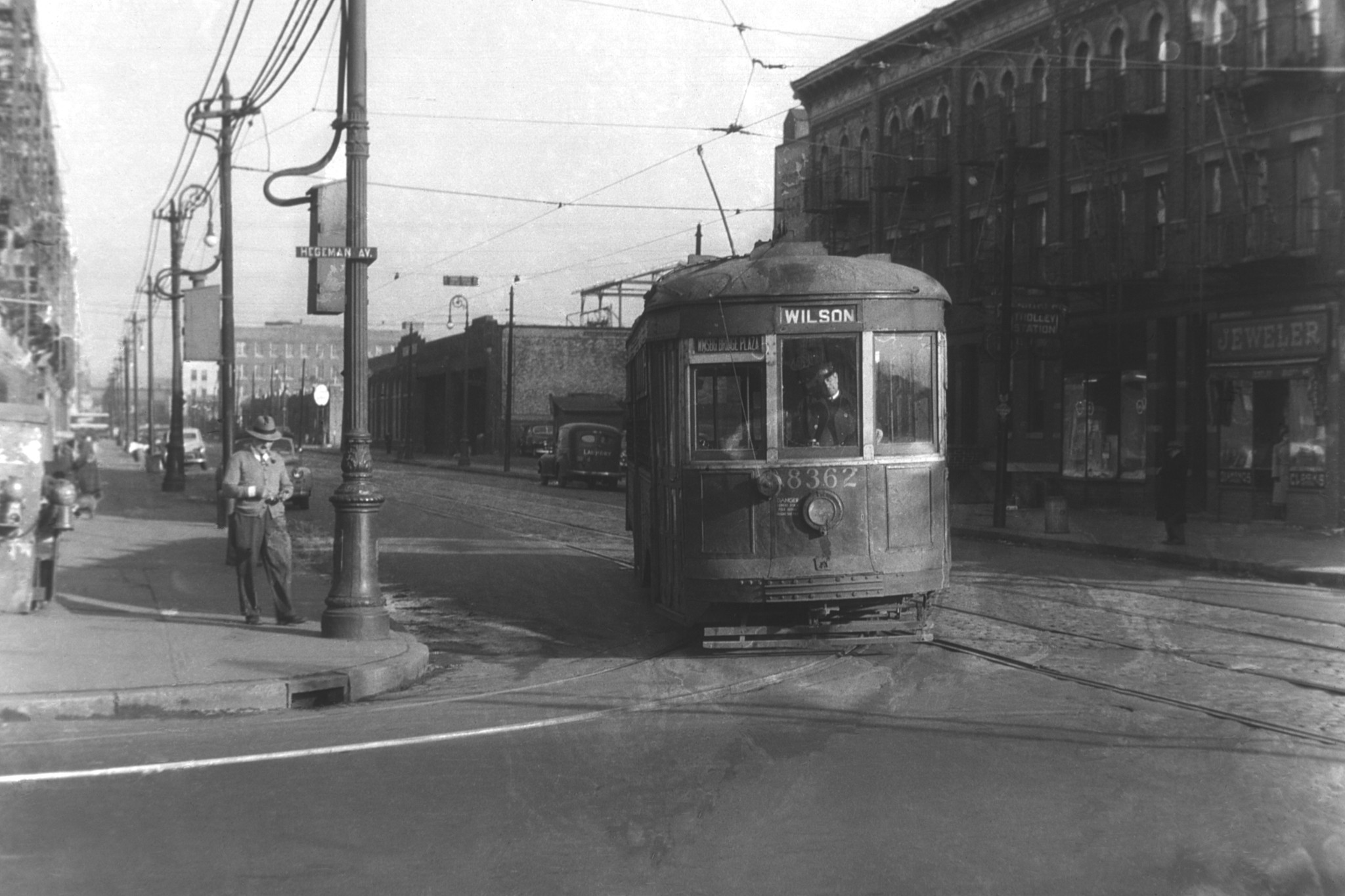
[623,149,950,658]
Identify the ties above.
[259,454,266,465]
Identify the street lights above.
[99,312,158,459]
[152,183,218,492]
[449,295,472,472]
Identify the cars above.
[135,424,210,472]
[227,438,310,509]
[536,422,623,486]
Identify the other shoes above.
[277,615,307,625]
[245,615,261,625]
[1162,538,1184,545]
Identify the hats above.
[244,415,282,441]
[1164,440,1182,452]
[815,360,836,380]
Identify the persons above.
[797,359,883,446]
[222,414,310,626]
